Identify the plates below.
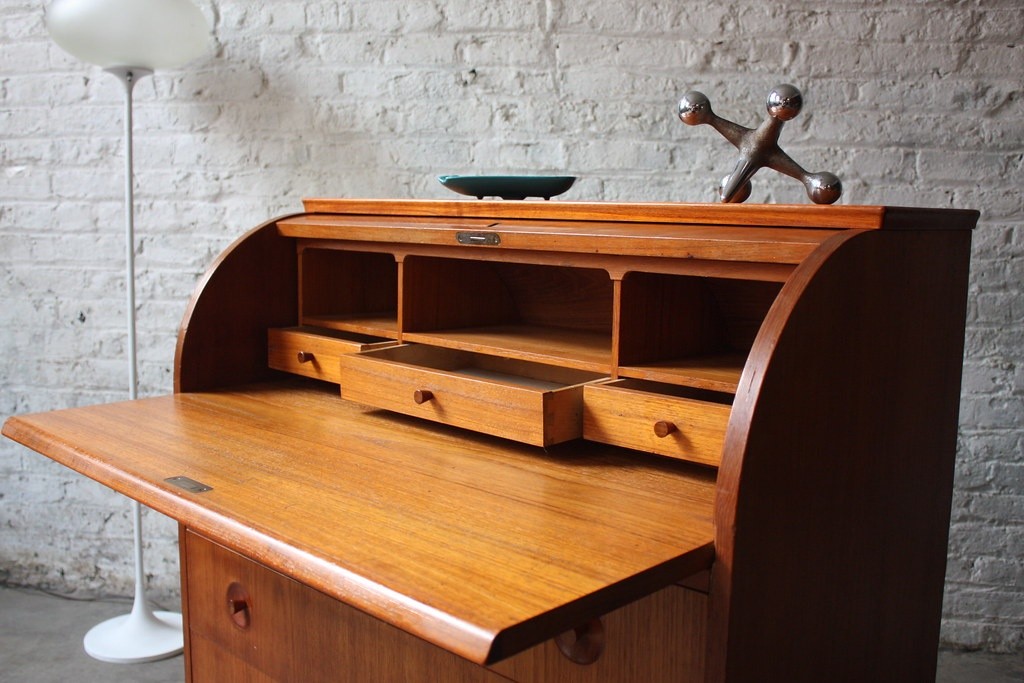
[439,173,577,200]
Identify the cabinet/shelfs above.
[1,198,982,683]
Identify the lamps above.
[42,0,211,664]
